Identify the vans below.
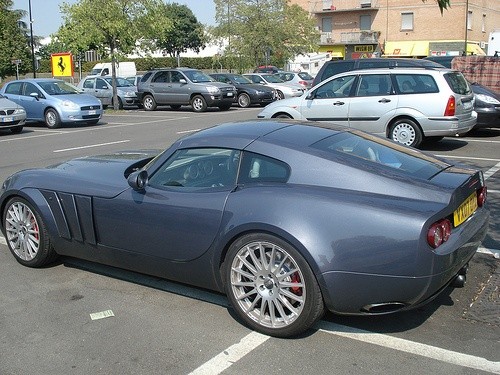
[87,61,137,80]
[309,58,500,134]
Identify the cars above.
[0,93,27,134]
[207,73,280,108]
[242,73,309,102]
[252,65,316,93]
[0,77,104,129]
[78,76,143,111]
[123,76,143,87]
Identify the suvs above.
[135,67,238,113]
[257,66,479,149]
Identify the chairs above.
[378,77,387,94]
[415,79,425,91]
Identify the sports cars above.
[0,116,491,339]
